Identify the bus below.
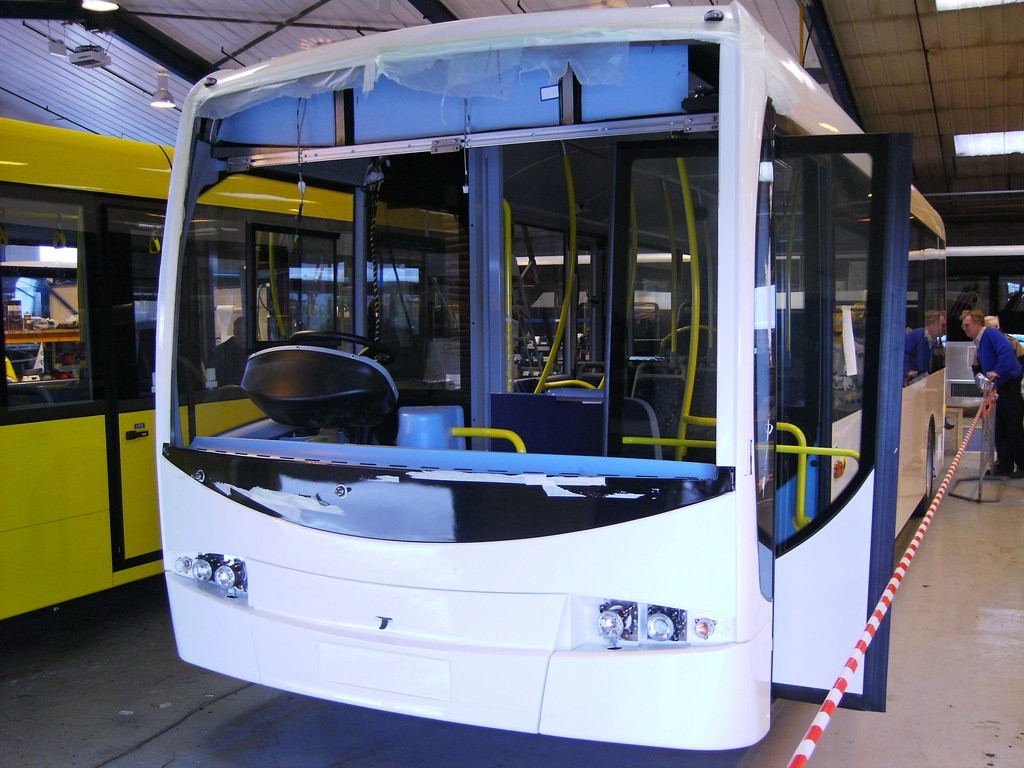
[0,120,460,621]
[103,3,948,750]
[259,246,1024,413]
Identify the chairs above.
[620,398,662,460]
[631,362,687,457]
[676,302,708,357]
[577,362,607,389]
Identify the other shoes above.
[985,461,1014,475]
[1011,470,1023,478]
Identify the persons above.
[207,315,248,388]
[902,309,948,382]
[959,310,1024,478]
